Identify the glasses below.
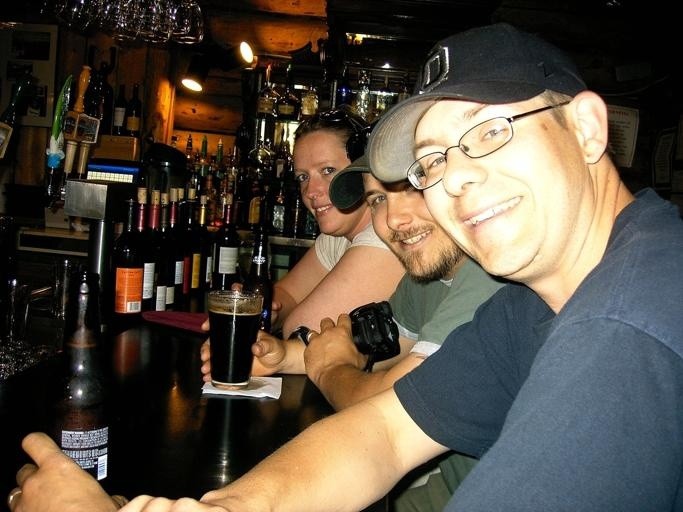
[297,111,363,130]
[346,116,383,163]
[403,98,572,192]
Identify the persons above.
[200,109,406,340]
[202,158,510,507]
[7,24,682,512]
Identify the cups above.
[4,277,32,351]
[207,290,263,389]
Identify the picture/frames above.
[0,21,58,128]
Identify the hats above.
[365,28,592,185]
[328,149,371,211]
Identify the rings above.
[6,487,20,505]
[306,330,319,343]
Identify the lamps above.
[205,33,254,73]
[182,38,210,92]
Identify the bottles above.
[47,271,114,489]
[113,84,128,134]
[0,80,29,172]
[127,84,141,136]
[89,62,112,127]
[242,224,272,333]
[169,134,235,191]
[0,223,18,300]
[247,60,412,239]
[110,187,241,317]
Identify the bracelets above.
[288,326,311,346]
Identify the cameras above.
[348,301,399,364]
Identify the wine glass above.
[38,0,206,47]
